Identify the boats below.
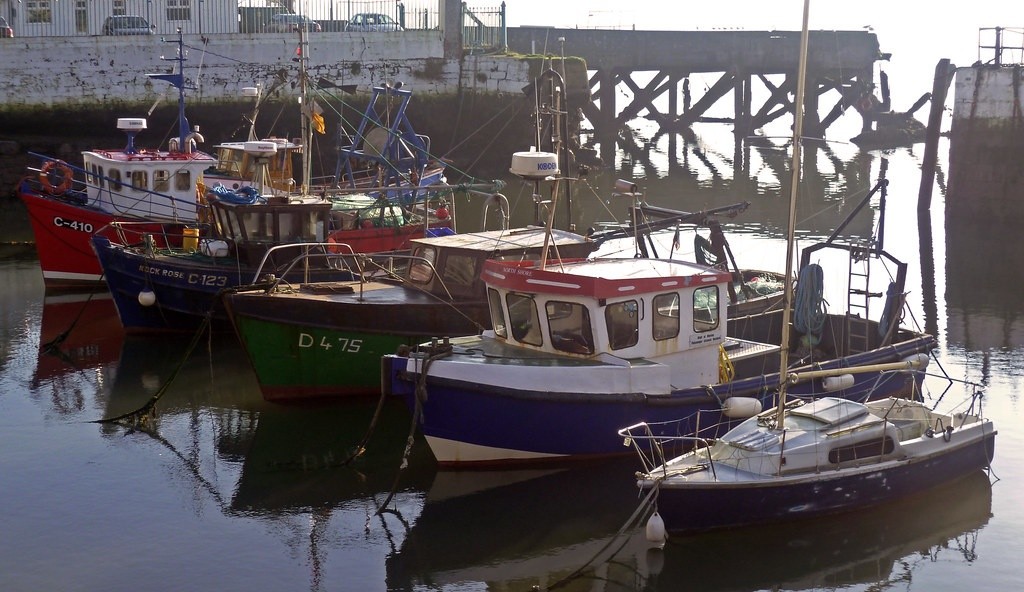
[88,16,458,339]
[223,36,806,390]
[617,0,1000,541]
[13,27,447,292]
[379,172,939,474]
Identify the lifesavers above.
[40,160,74,195]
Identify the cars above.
[344,13,404,32]
[101,14,156,36]
[259,13,321,33]
[0,15,13,38]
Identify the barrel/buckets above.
[182,227,200,253]
